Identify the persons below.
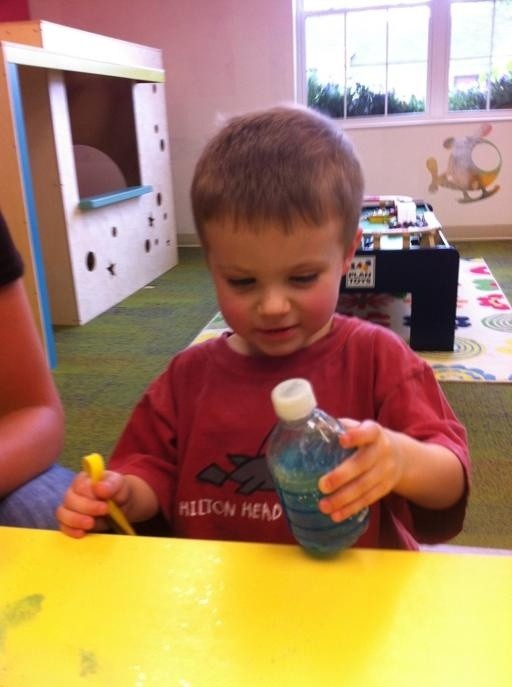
[55,102,471,552]
[0,210,79,531]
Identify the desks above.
[340,196,460,352]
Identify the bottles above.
[266,378,370,560]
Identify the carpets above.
[183,258,512,384]
